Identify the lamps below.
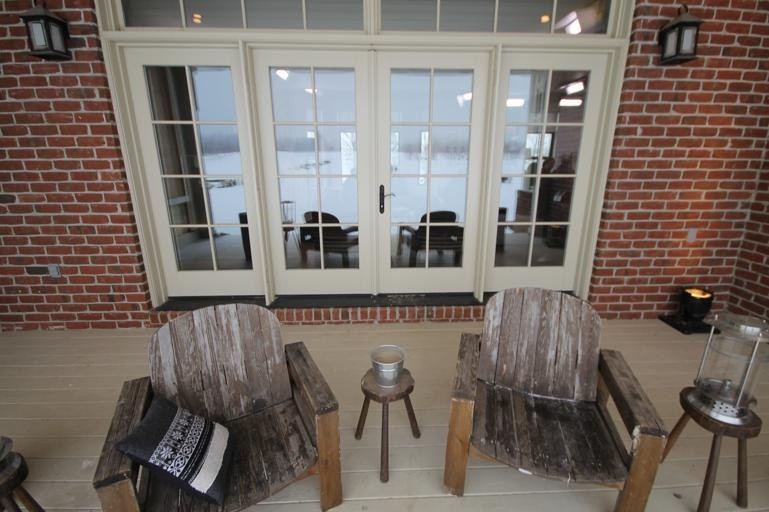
[14,0,72,60]
[658,3,704,65]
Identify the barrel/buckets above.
[371,344,405,389]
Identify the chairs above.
[443,287,667,512]
[91,304,343,512]
[397,211,463,267]
[300,211,358,268]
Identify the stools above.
[660,388,762,512]
[354,368,420,483]
[0,448,47,512]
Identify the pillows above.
[116,394,235,507]
